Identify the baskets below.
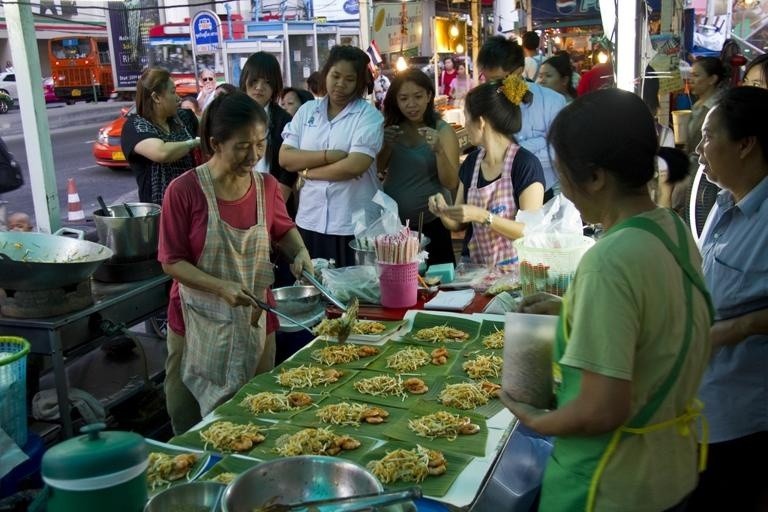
[514,232,597,297]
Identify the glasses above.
[478,65,520,85]
[203,77,214,82]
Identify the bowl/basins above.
[270,285,322,315]
[142,481,228,512]
[219,454,385,512]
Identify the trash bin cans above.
[671,110,693,145]
[0,336,31,449]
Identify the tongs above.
[260,487,422,512]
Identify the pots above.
[348,236,431,266]
[0,227,113,291]
[92,202,162,263]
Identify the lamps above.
[450,27,459,38]
[397,57,407,71]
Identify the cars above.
[0,92,14,113]
[93,88,200,168]
[0,73,44,99]
[407,56,442,77]
[455,54,471,71]
[43,72,58,103]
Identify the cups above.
[501,311,560,409]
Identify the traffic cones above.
[65,177,89,224]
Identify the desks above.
[326,286,493,322]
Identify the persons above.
[694,84,768,511]
[158,89,315,436]
[499,90,712,512]
[118,30,767,282]
[8,211,33,233]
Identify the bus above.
[150,37,215,95]
[48,36,113,105]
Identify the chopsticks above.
[374,219,419,264]
